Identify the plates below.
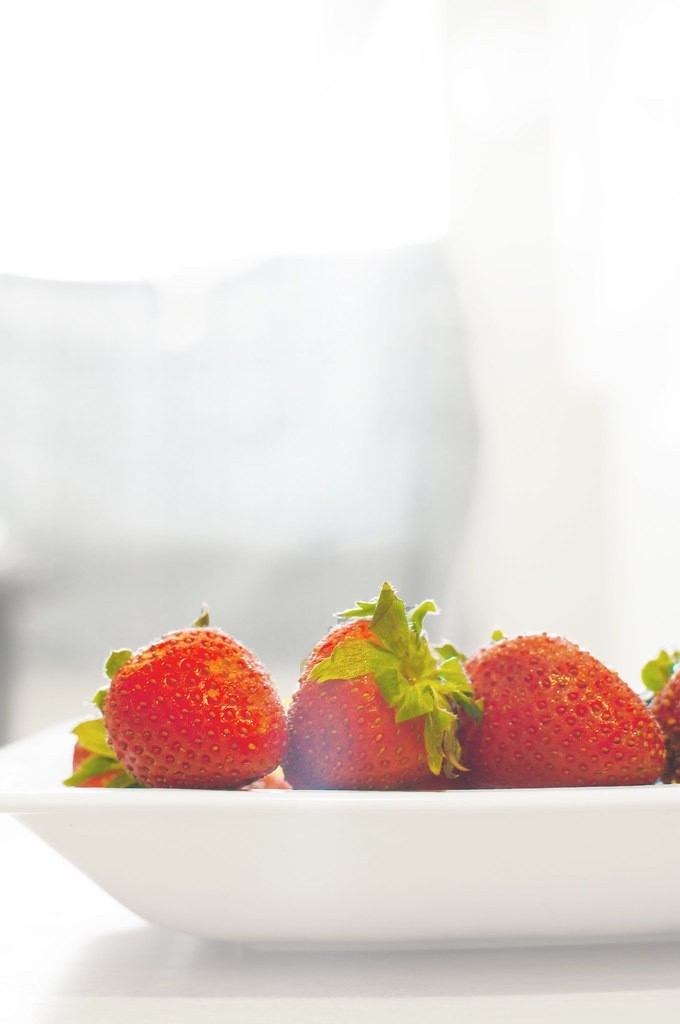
[0,715,680,953]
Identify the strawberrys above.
[63,582,680,794]
